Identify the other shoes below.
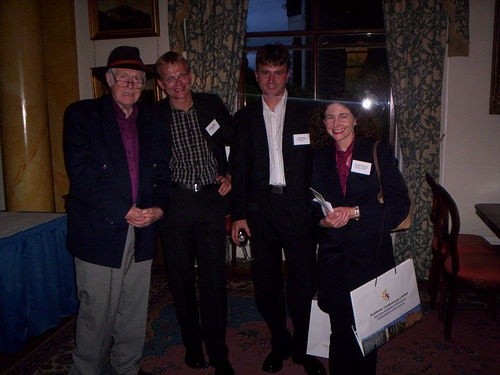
[209,356,235,375]
[184,342,206,368]
[262,340,292,373]
[291,352,327,375]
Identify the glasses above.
[112,71,144,89]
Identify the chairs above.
[424,173,500,342]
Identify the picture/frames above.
[87,0,160,40]
[90,64,162,103]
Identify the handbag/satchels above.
[349,204,424,357]
[376,138,413,232]
[306,291,331,359]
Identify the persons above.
[308,94,411,375]
[152,51,235,375]
[230,43,327,375]
[62,45,170,375]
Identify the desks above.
[0,211,79,360]
[474,203,500,239]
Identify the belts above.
[269,185,287,195]
[172,183,221,192]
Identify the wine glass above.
[237,230,255,262]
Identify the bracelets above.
[355,206,359,220]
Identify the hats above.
[90,46,161,84]
